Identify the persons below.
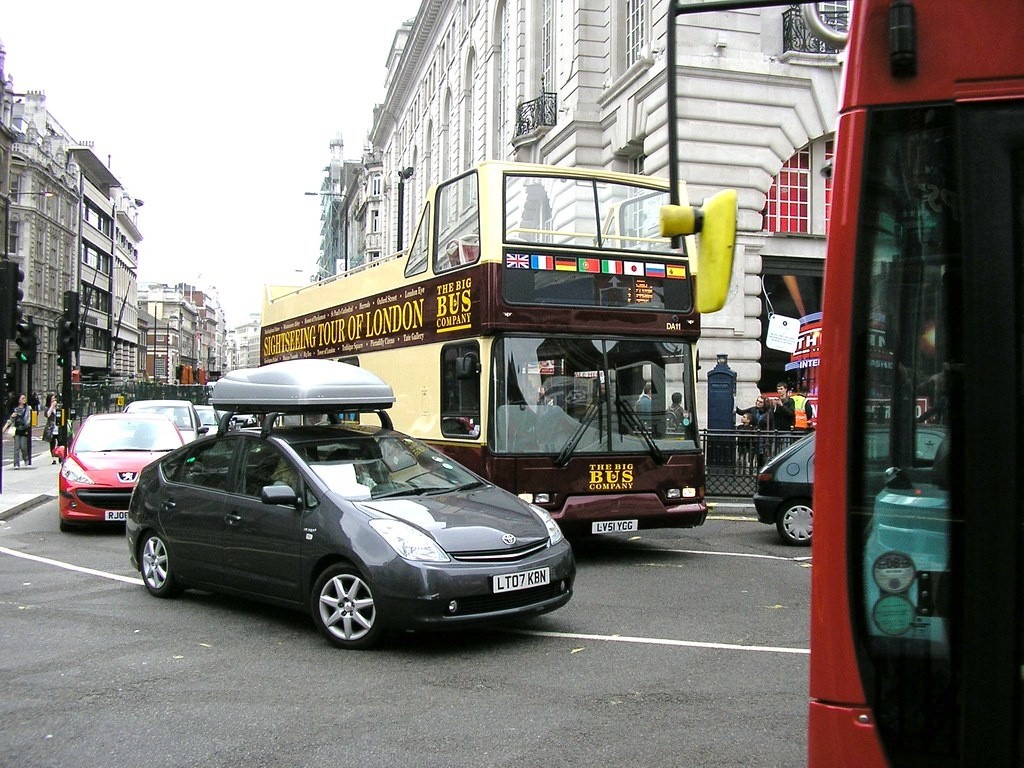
[344,450,377,493]
[2,391,78,470]
[920,360,952,426]
[635,383,812,474]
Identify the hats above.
[800,386,811,393]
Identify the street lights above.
[305,192,349,275]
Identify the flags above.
[506,253,685,279]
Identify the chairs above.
[446,232,480,267]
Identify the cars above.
[53,412,186,533]
[123,400,228,448]
[125,357,577,649]
[753,422,953,545]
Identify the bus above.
[260,159,742,535]
[809,1,1024,768]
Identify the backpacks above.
[666,406,681,428]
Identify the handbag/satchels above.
[765,448,771,457]
[2,417,17,437]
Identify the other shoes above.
[15,465,18,468]
[25,460,28,466]
[52,461,56,464]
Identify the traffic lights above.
[0,261,25,339]
[57,358,66,366]
[57,319,77,354]
[14,321,37,365]
